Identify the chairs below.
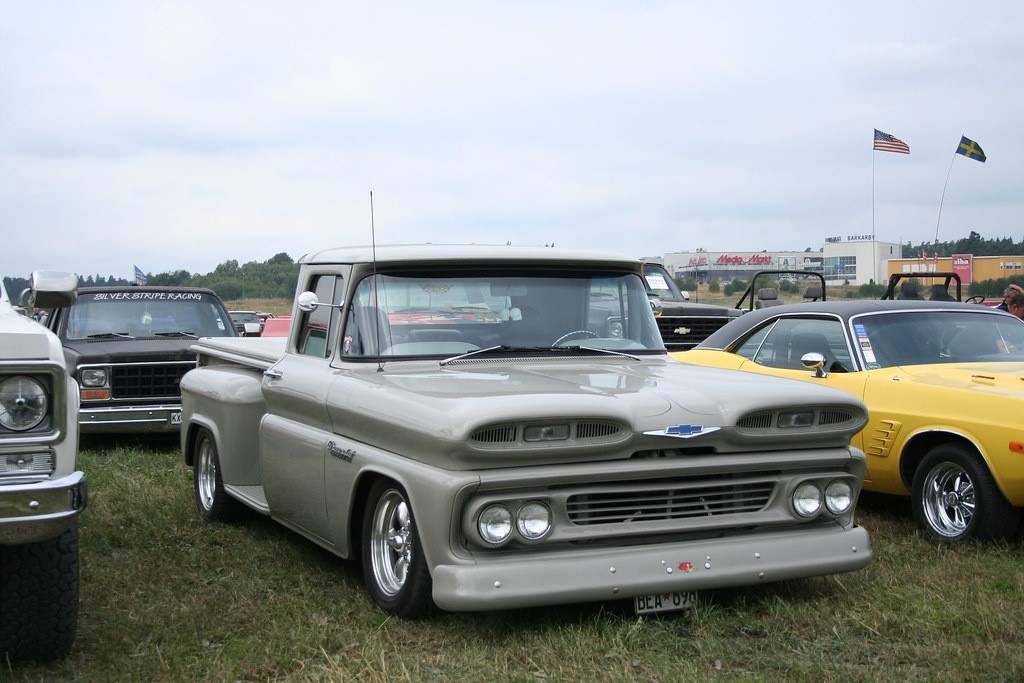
[897,282,925,300]
[409,329,462,342]
[946,325,1000,360]
[68,318,112,338]
[755,287,784,309]
[803,285,823,303]
[929,284,956,301]
[133,316,179,333]
[788,333,849,373]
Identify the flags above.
[956,136,986,163]
[135,266,147,285]
[874,130,910,154]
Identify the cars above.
[176,247,874,617]
[228,284,518,349]
[0,265,88,665]
[29,282,268,443]
[590,260,753,355]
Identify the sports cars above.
[668,295,1024,548]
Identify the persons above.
[997,284,1024,321]
[780,259,788,270]
